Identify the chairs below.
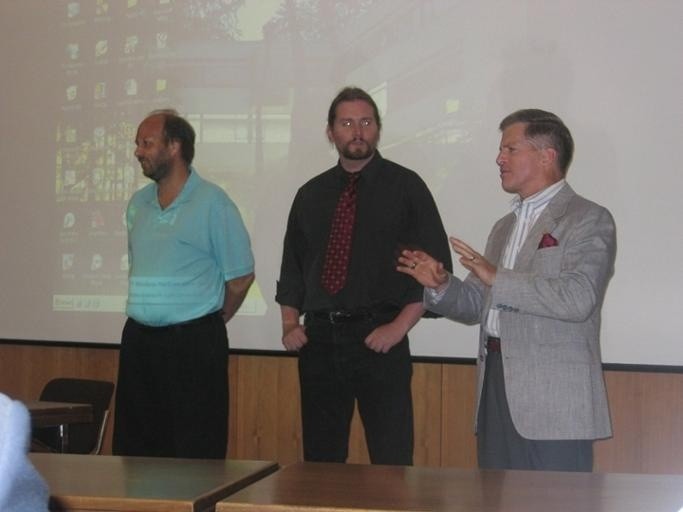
[30,378,115,454]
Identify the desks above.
[10,396,92,453]
[24,454,279,512]
[216,461,683,512]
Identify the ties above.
[320,177,361,298]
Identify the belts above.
[308,310,389,322]
[485,337,502,353]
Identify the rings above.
[410,262,416,269]
[470,256,475,261]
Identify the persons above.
[394,108,616,474]
[111,107,257,459]
[276,87,452,463]
